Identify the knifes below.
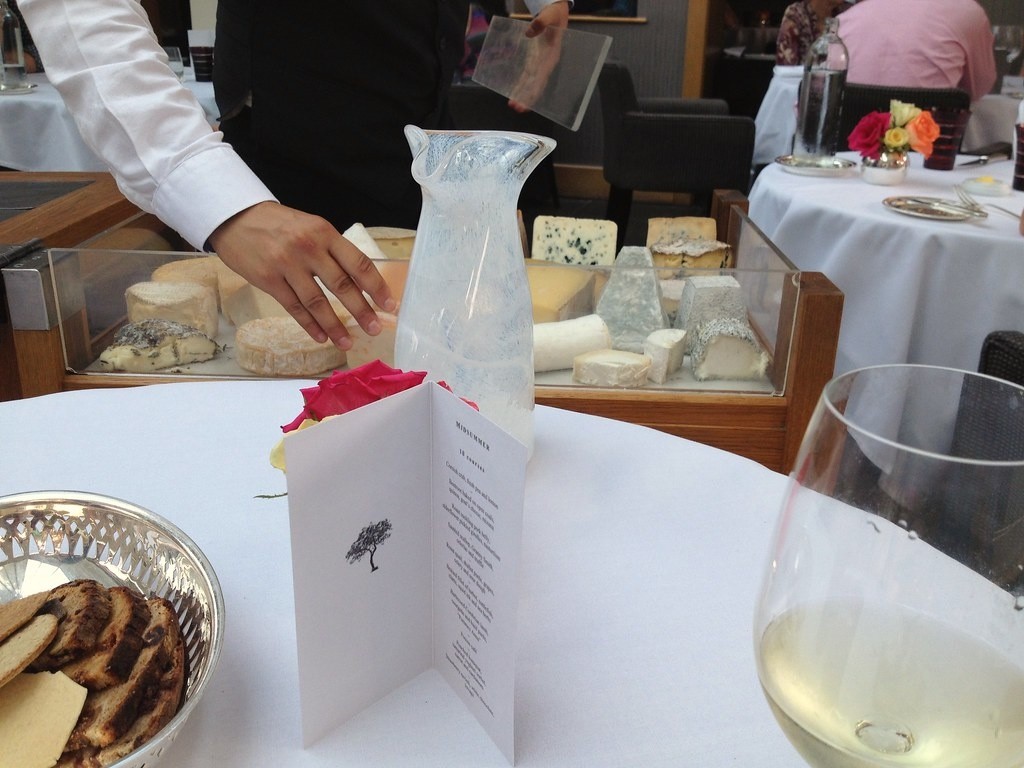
[959,151,1008,169]
[897,195,988,218]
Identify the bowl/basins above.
[0,490,226,768]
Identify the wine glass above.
[992,25,1024,87]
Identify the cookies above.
[0,589,88,768]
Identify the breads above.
[23,578,185,768]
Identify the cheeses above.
[525,216,769,388]
[100,255,398,375]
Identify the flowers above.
[254,362,482,500]
[846,99,939,160]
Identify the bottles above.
[393,124,556,465]
[0,0,31,95]
[791,17,850,166]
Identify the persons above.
[827,0,1002,102]
[774,0,854,66]
[15,0,569,350]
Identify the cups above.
[162,47,184,81]
[755,360,1022,768]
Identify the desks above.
[0,71,221,172]
[736,65,1024,531]
[0,380,1024,768]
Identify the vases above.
[860,146,909,185]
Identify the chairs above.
[596,56,756,259]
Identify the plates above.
[774,153,858,175]
[881,196,975,220]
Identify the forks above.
[953,184,1021,221]
